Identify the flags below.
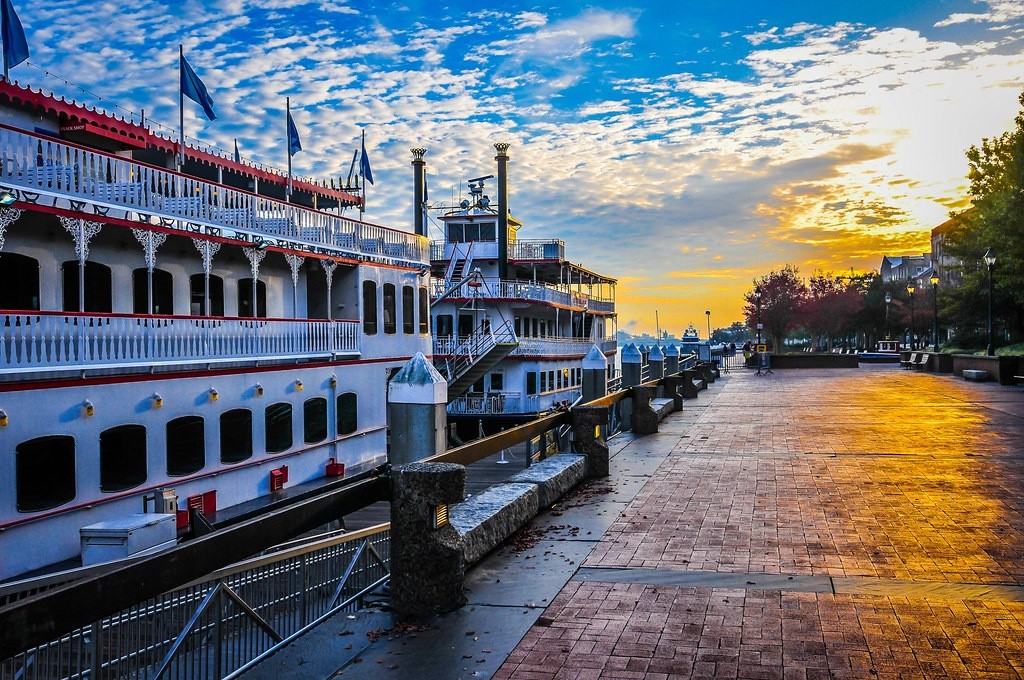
[288,112,302,157]
[235,146,240,162]
[359,147,374,185]
[1,0,29,69]
[180,54,217,121]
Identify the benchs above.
[963,369,987,382]
[803,348,868,354]
[912,354,930,371]
[901,352,918,370]
[391,406,613,613]
[16,162,404,257]
[681,365,709,397]
[632,376,685,434]
[704,360,720,382]
[1014,376,1024,379]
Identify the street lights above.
[929,269,940,352]
[755,286,762,366]
[885,290,892,349]
[705,311,710,362]
[906,280,916,351]
[983,246,996,356]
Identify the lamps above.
[296,378,303,391]
[330,377,337,387]
[254,241,269,251]
[210,388,218,401]
[0,409,8,427]
[153,391,163,408]
[256,383,264,396]
[82,400,94,417]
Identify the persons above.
[741,341,757,369]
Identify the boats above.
[0,74,433,583]
[680,322,700,360]
[410,142,621,419]
